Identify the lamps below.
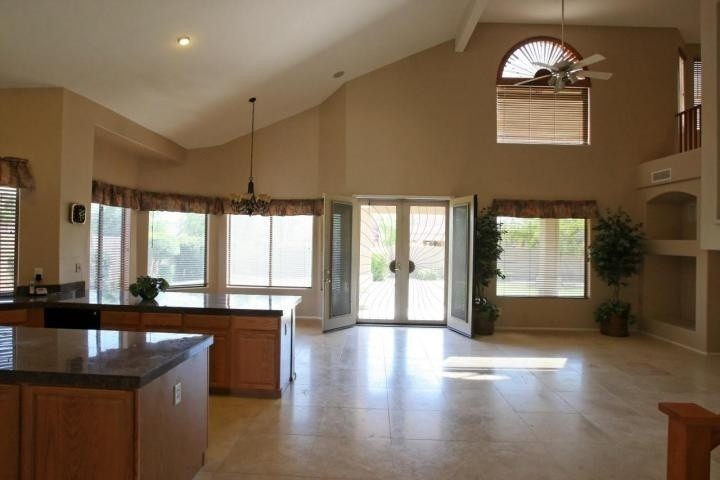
[229,98,272,216]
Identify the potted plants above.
[130,275,170,302]
[587,207,648,338]
[474,208,511,335]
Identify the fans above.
[514,0,613,94]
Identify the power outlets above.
[34,267,44,280]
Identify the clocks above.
[70,204,87,225]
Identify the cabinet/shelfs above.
[101,310,280,396]
[0,384,133,480]
